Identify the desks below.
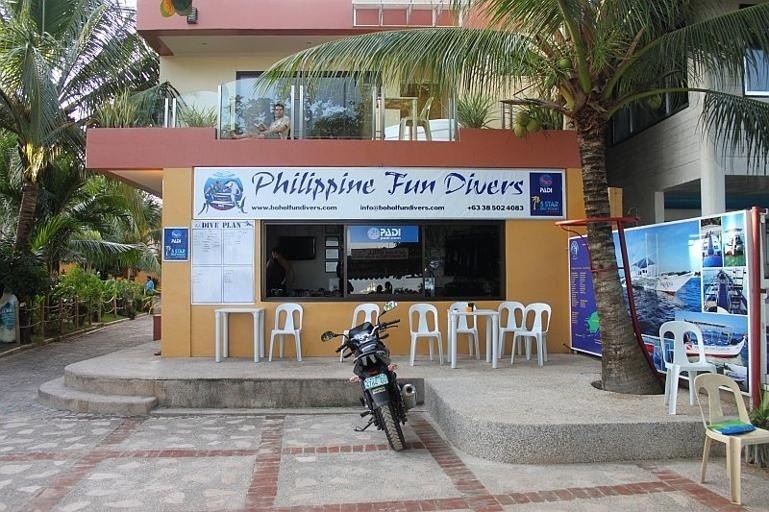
[376,96,417,140]
[213,307,266,363]
[446,309,500,369]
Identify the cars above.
[702,233,723,267]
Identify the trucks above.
[724,234,743,256]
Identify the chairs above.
[450,302,482,361]
[267,302,304,363]
[658,320,721,416]
[510,302,552,368]
[399,96,434,141]
[339,302,381,362]
[693,373,769,505]
[497,301,530,361]
[408,304,444,367]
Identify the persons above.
[145,275,155,297]
[253,103,291,140]
[376,285,383,294]
[339,271,354,297]
[384,281,392,294]
[265,246,288,296]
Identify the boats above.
[618,231,695,294]
[726,371,745,382]
[683,318,746,359]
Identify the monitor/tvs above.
[276,235,316,260]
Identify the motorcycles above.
[320,301,417,452]
